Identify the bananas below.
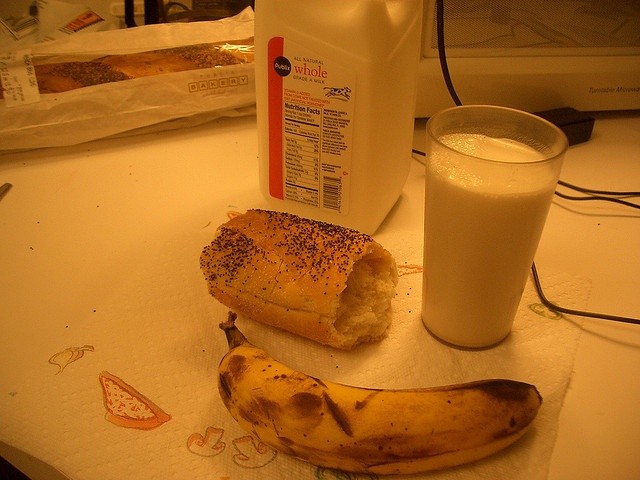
[215,312,543,476]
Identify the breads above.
[200,208,400,353]
[36,36,256,94]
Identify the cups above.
[421,104,567,349]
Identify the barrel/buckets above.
[254,0,423,235]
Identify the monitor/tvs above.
[414,1,640,120]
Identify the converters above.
[526,105,596,146]
